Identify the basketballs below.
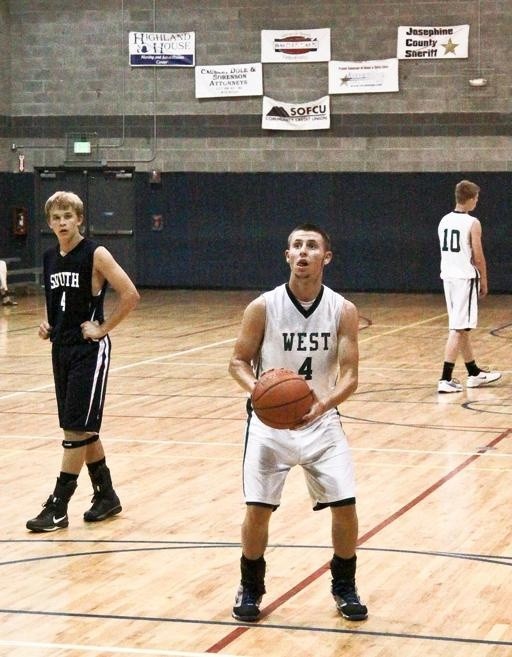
[252,368,313,429]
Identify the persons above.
[225,220,372,622]
[1,258,19,306]
[433,178,504,396]
[25,188,144,533]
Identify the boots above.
[26,477,77,531]
[84,465,122,522]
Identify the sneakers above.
[438,378,463,393]
[467,369,501,387]
[233,580,265,620]
[330,579,367,621]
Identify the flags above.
[259,93,333,135]
[194,61,265,99]
[394,22,470,60]
[258,24,335,64]
[326,56,402,95]
[126,28,197,70]
